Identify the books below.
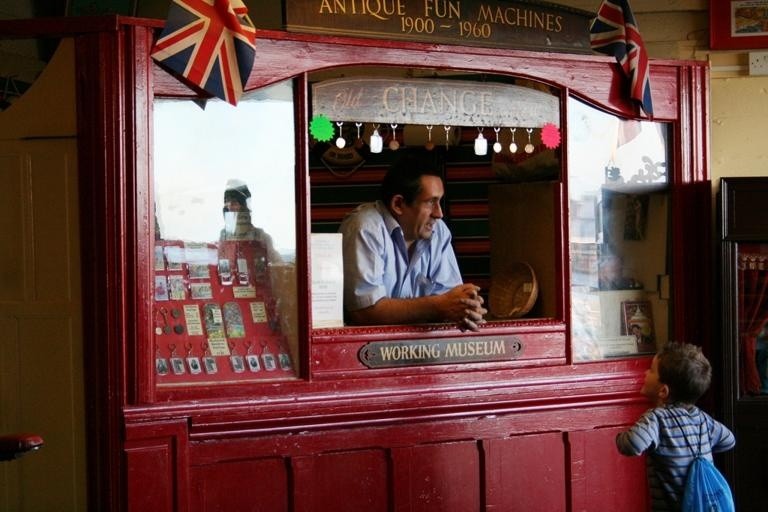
[621,300,658,353]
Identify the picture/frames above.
[708,0,768,50]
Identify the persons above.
[213,185,281,289]
[614,337,739,511]
[631,325,647,345]
[337,156,491,332]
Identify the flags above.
[585,0,659,122]
[152,0,256,106]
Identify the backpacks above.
[664,408,737,512]
